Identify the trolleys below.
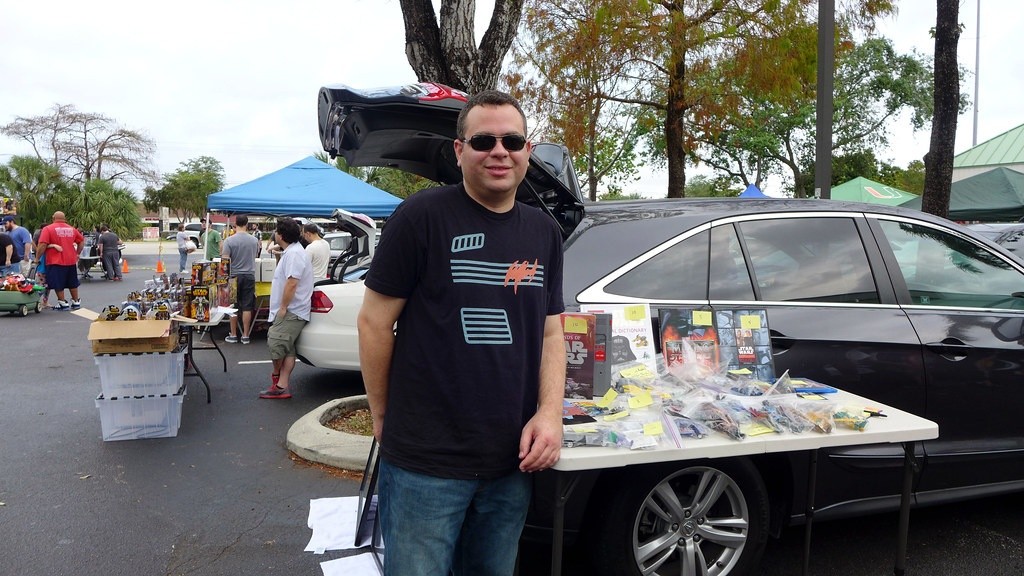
[0,260,44,317]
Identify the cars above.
[315,80,1024,575]
[293,208,397,373]
[166,235,177,240]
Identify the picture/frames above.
[355,436,380,546]
[370,502,385,575]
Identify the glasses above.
[463,134,527,151]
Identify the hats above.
[0,216,14,224]
[200,218,212,225]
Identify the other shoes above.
[42,304,54,309]
[180,270,188,274]
[119,278,122,281]
[109,279,113,281]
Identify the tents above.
[739,166,1024,221]
[204,157,403,259]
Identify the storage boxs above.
[254,258,276,282]
[69,257,231,442]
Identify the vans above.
[184,223,227,249]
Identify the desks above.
[550,385,939,576]
[200,282,274,341]
[180,304,235,405]
[79,255,107,283]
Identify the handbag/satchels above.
[184,239,197,254]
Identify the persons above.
[177,226,190,273]
[0,211,84,310]
[199,218,236,261]
[252,223,262,258]
[94,226,122,281]
[222,216,259,344]
[265,221,330,281]
[259,218,314,398]
[358,90,566,576]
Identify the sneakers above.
[53,300,70,311]
[241,336,250,344]
[70,298,82,310]
[272,373,280,387]
[225,333,238,343]
[259,386,291,398]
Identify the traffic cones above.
[120,259,130,273]
[155,263,165,273]
